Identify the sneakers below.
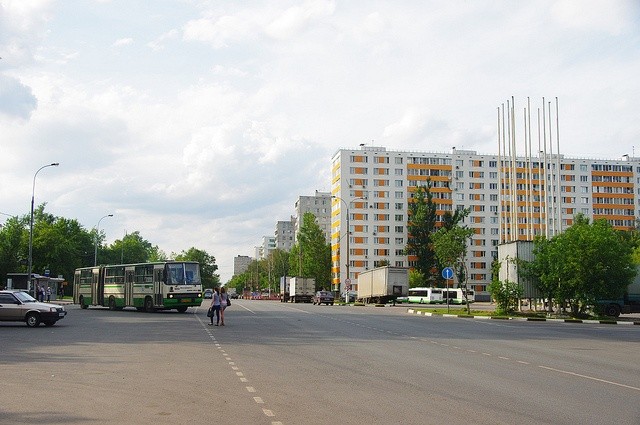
[214,324,218,326]
[208,323,213,326]
[220,323,224,326]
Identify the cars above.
[1,290,68,326]
[203,288,214,299]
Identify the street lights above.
[328,195,366,305]
[29,163,60,298]
[93,214,114,266]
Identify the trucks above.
[289,277,314,303]
[279,276,293,303]
[227,287,240,299]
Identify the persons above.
[47,285,51,301]
[220,288,228,326]
[208,287,220,326]
[41,285,45,302]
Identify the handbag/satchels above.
[207,307,214,317]
[226,295,231,306]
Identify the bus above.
[311,290,334,306]
[73,260,203,313]
[397,288,444,305]
[458,288,476,305]
[416,287,462,305]
